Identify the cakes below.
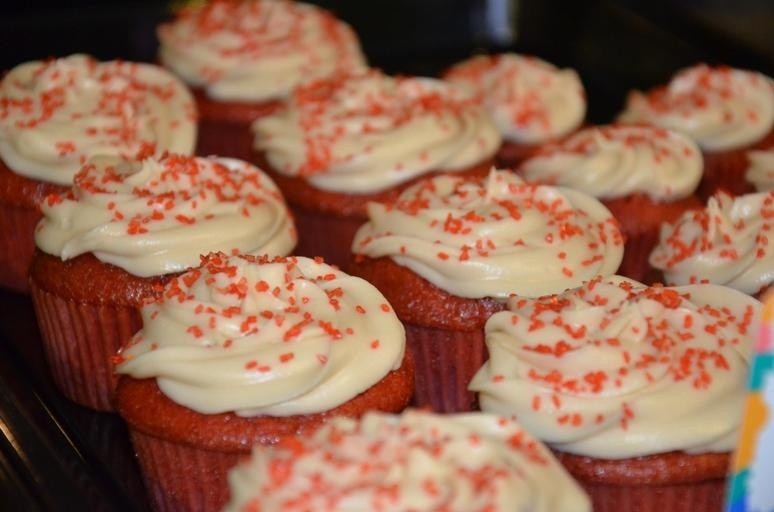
[1,0,774,512]
